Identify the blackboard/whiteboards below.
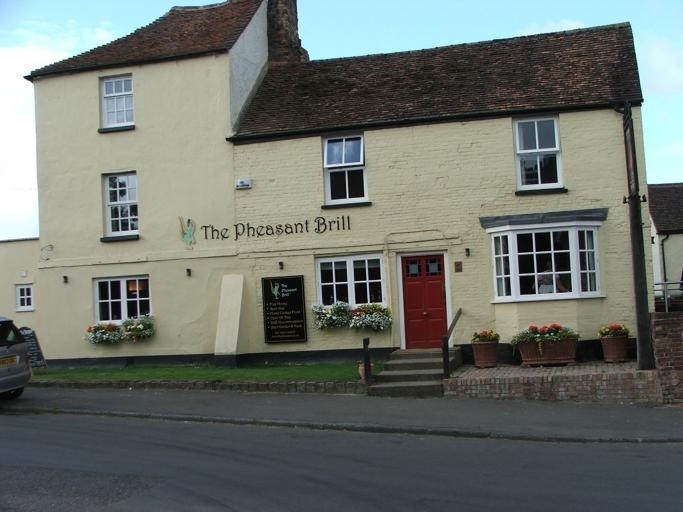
[19,327,47,368]
[261,275,307,343]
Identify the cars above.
[0,316,32,397]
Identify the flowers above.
[312,300,393,333]
[597,322,630,337]
[471,328,498,342]
[82,311,157,346]
[512,323,579,351]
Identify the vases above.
[518,338,577,365]
[472,343,498,368]
[600,335,630,363]
